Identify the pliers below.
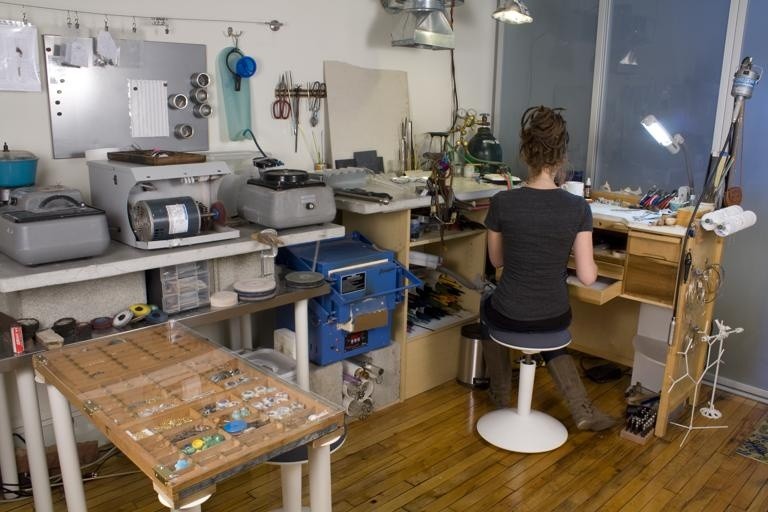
[311,81,326,126]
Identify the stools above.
[476,323,572,454]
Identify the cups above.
[561,180,587,198]
[314,163,327,170]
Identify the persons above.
[477,102,621,432]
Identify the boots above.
[547,354,616,431]
[478,339,513,408]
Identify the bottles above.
[573,170,584,182]
[584,177,592,199]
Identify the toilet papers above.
[700,205,743,231]
[714,210,756,237]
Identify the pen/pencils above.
[638,185,677,210]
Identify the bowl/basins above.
[669,202,686,212]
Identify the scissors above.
[273,74,291,119]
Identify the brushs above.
[701,152,757,237]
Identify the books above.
[585,196,661,226]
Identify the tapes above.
[54,304,168,339]
[16,318,40,333]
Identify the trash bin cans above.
[456,323,490,390]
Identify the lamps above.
[640,114,695,205]
[450,1,534,135]
